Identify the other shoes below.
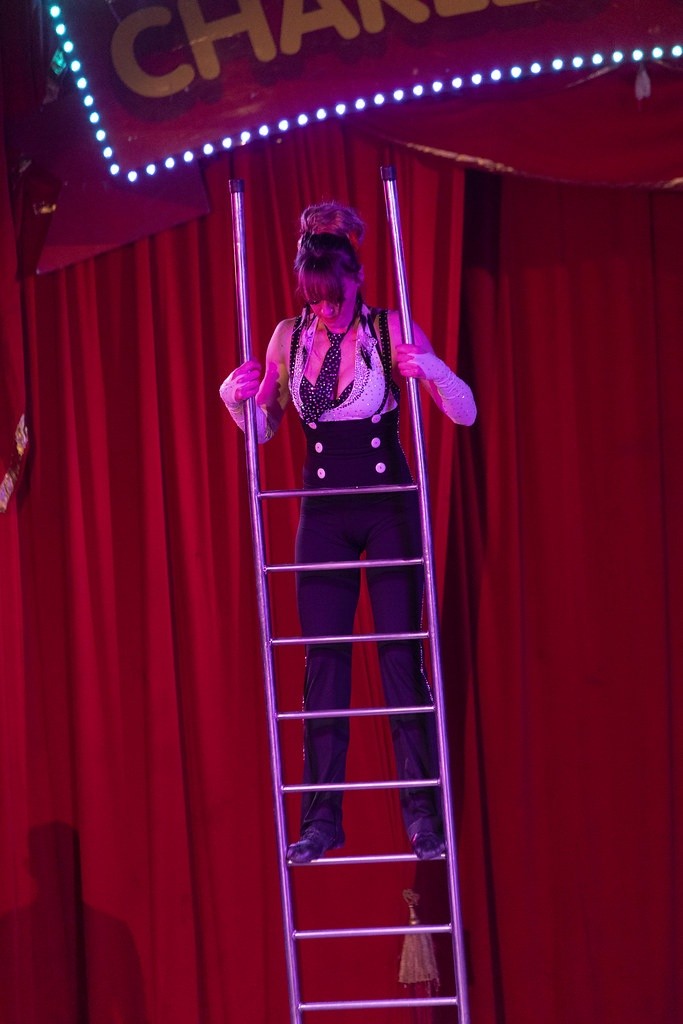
[287,825,346,864]
[410,831,446,860]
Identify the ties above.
[303,314,357,424]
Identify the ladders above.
[230,162,472,1024]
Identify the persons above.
[220,204,476,862]
